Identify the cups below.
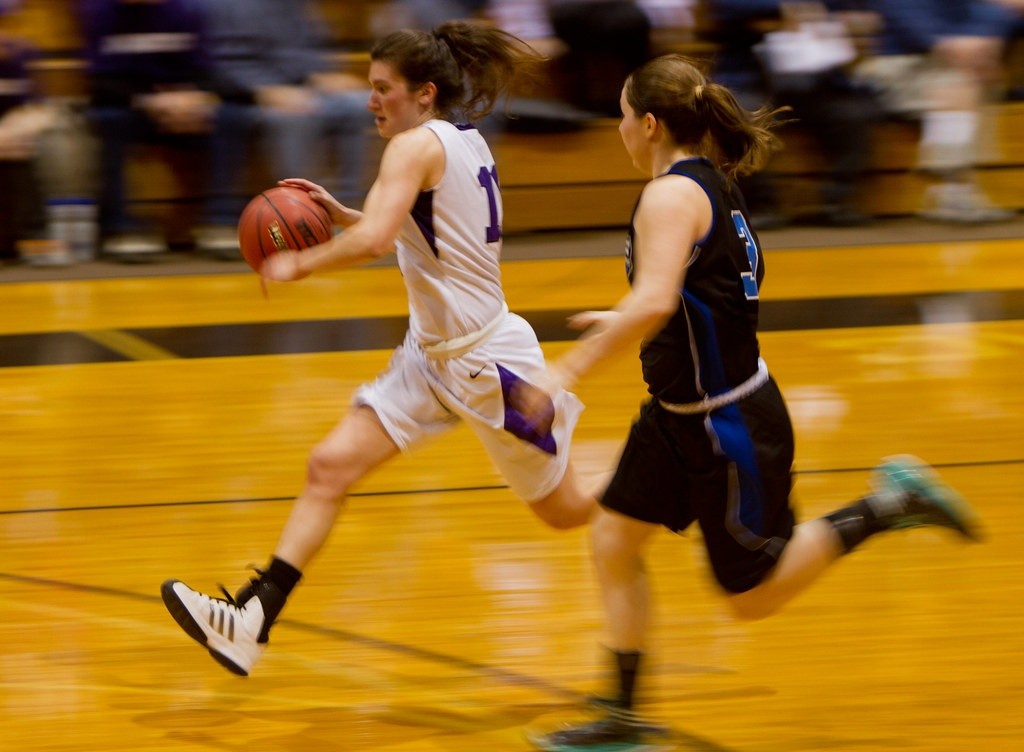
[48,198,98,262]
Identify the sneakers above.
[883,461,975,540]
[160,578,270,678]
[540,720,642,752]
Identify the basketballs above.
[238,187,333,282]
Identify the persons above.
[160,21,616,678]
[512,56,990,752]
[0,1,1024,270]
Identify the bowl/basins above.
[103,243,167,262]
[200,238,244,261]
